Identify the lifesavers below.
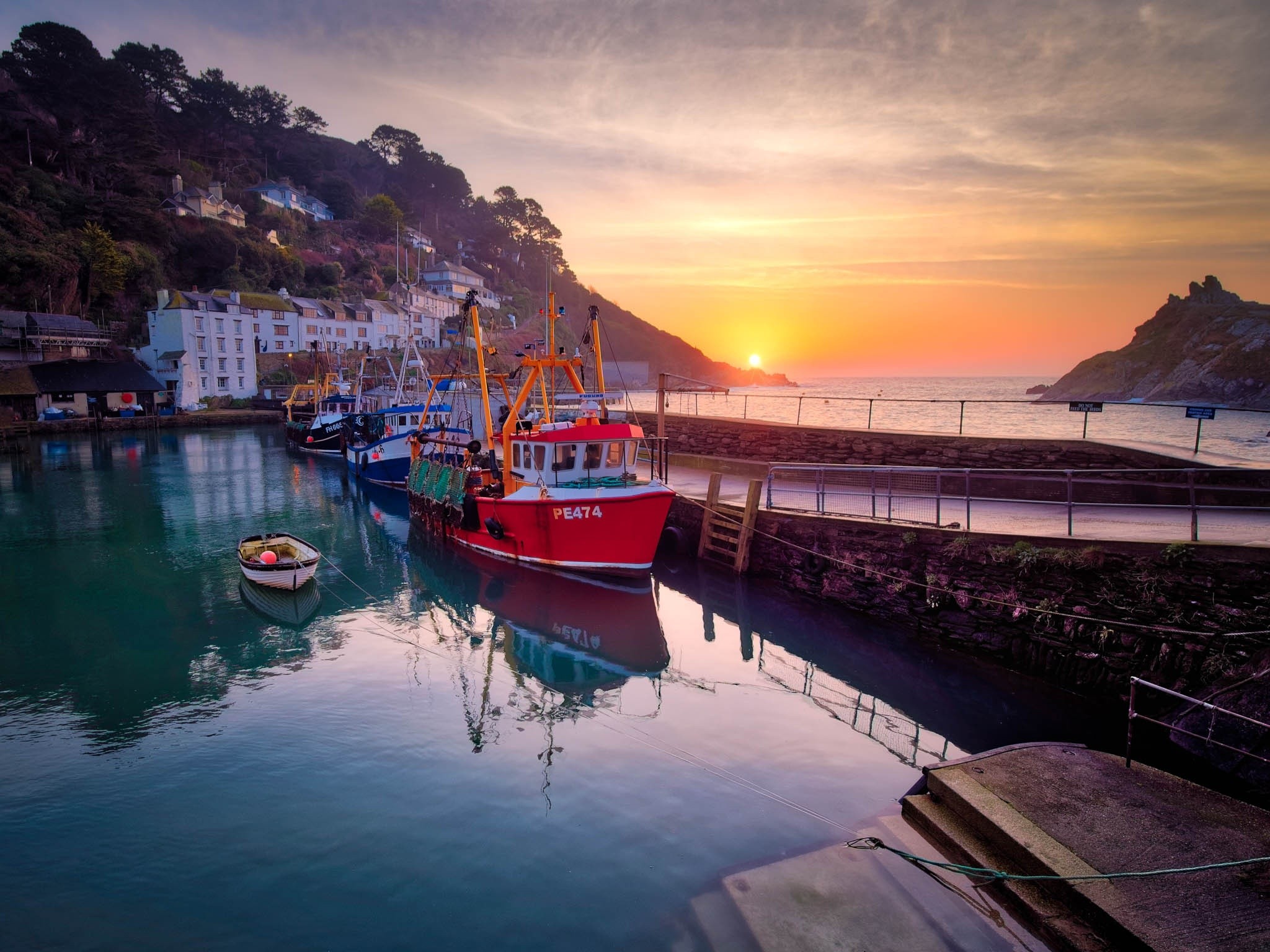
[385,425,392,437]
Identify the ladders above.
[697,473,763,574]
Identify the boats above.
[345,333,476,493]
[281,327,386,459]
[237,570,322,631]
[237,532,322,591]
[404,285,676,586]
